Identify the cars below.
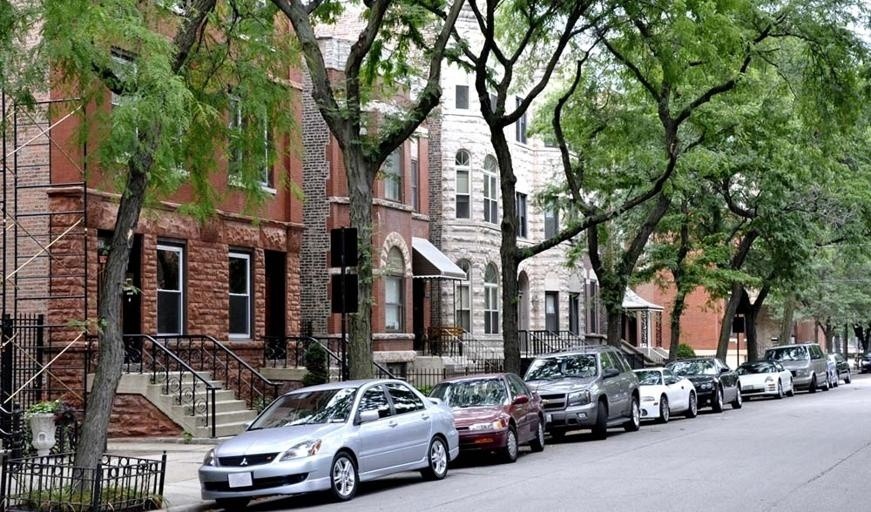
[197,378,460,511]
[826,351,853,387]
[664,357,743,413]
[861,351,871,372]
[426,372,548,463]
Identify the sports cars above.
[633,366,699,423]
[733,359,795,399]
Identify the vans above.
[762,339,830,394]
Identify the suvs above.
[523,344,641,439]
